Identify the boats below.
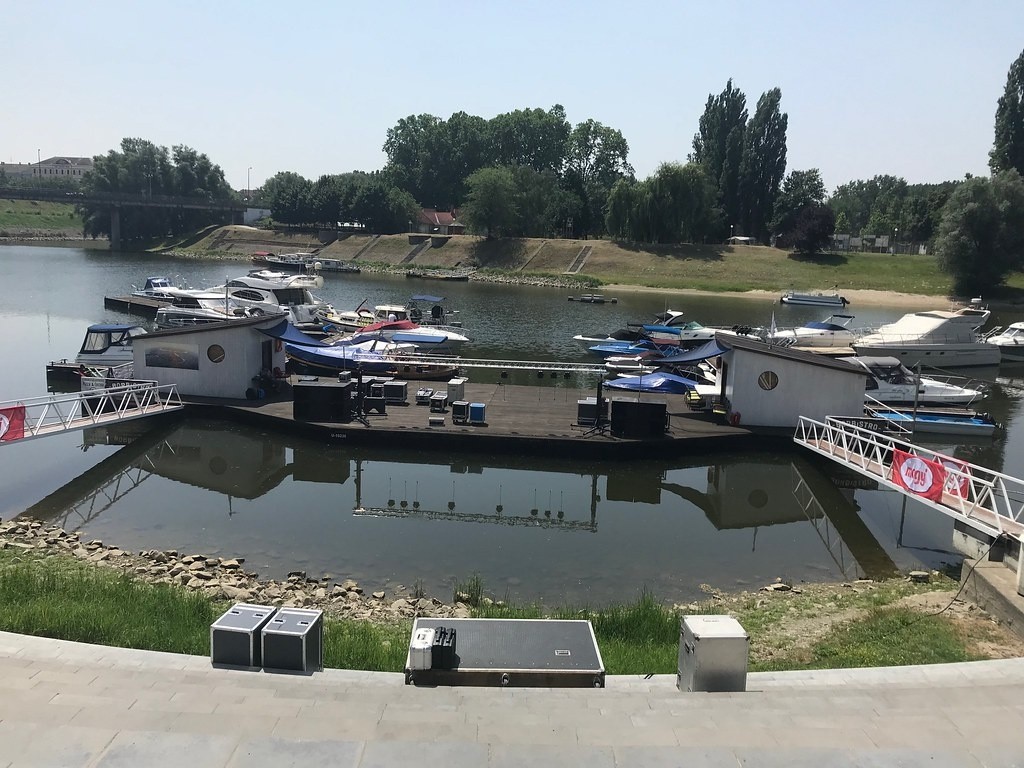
[70,249,471,377]
[782,292,851,308]
[850,298,1003,366]
[830,356,989,403]
[984,322,1024,361]
[569,304,860,393]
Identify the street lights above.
[246,166,253,207]
[893,227,900,255]
[731,224,733,245]
[37,149,42,184]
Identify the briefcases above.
[410,628,435,670]
[442,628,456,669]
[432,627,446,669]
